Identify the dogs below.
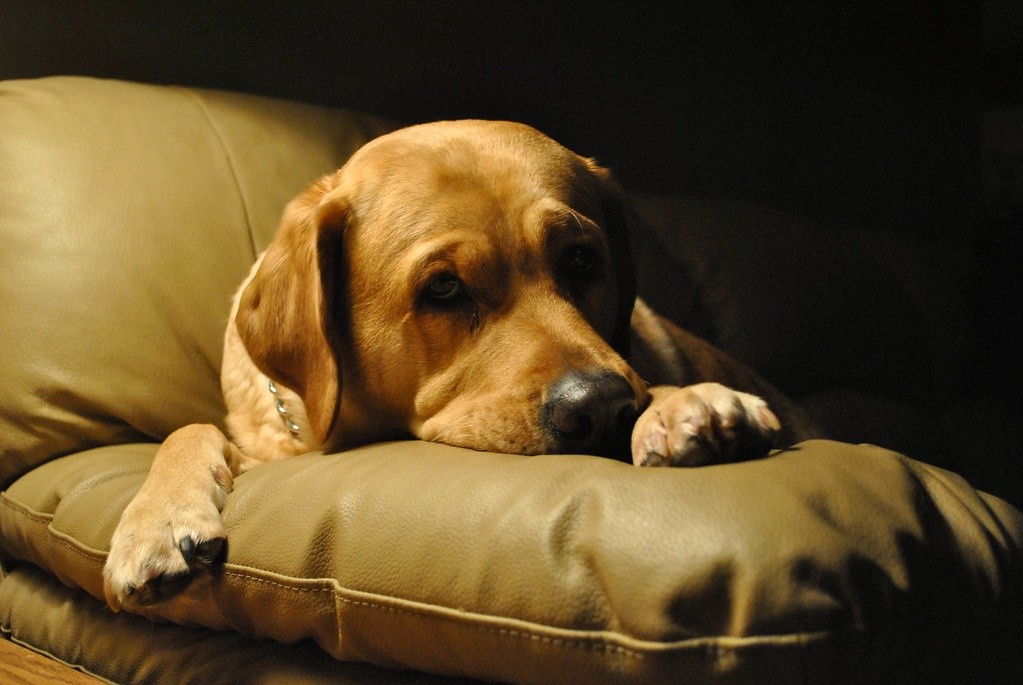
[97,116,1023,617]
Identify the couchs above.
[0,52,1023,685]
